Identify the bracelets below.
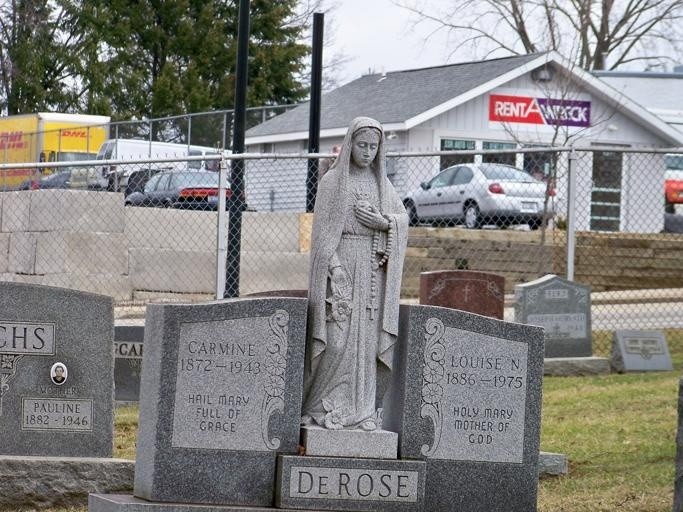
[329,265,342,275]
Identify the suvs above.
[124,165,248,211]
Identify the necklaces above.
[365,214,394,321]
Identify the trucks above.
[0,111,111,191]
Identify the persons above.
[319,146,341,181]
[298,115,410,435]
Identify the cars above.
[664,153,683,214]
[400,162,558,230]
[19,167,102,190]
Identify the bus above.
[96,139,232,192]
